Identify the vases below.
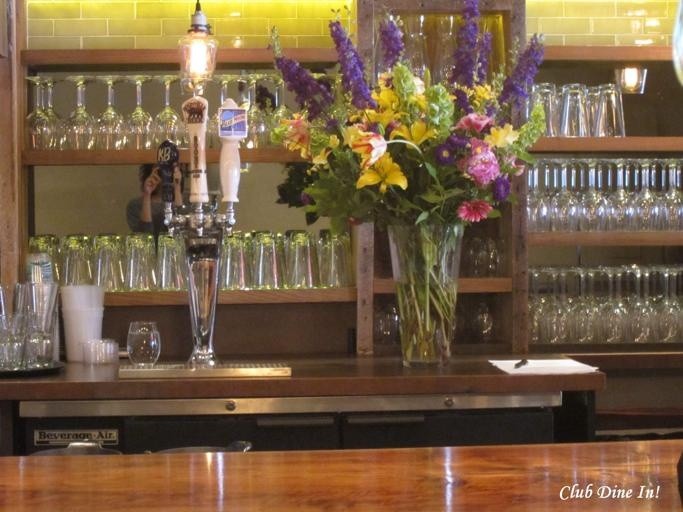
[388,222,464,370]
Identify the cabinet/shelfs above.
[22,42,682,373]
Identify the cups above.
[0,282,59,372]
[28,231,352,291]
[82,336,120,366]
[62,286,106,360]
[125,320,162,370]
[525,81,627,138]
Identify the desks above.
[0,437,681,511]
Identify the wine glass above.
[24,73,291,148]
[529,264,683,343]
[525,155,682,231]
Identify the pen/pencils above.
[515,358,529,368]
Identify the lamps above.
[609,62,649,95]
[142,0,263,232]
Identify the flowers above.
[255,18,540,356]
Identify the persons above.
[126,164,184,253]
